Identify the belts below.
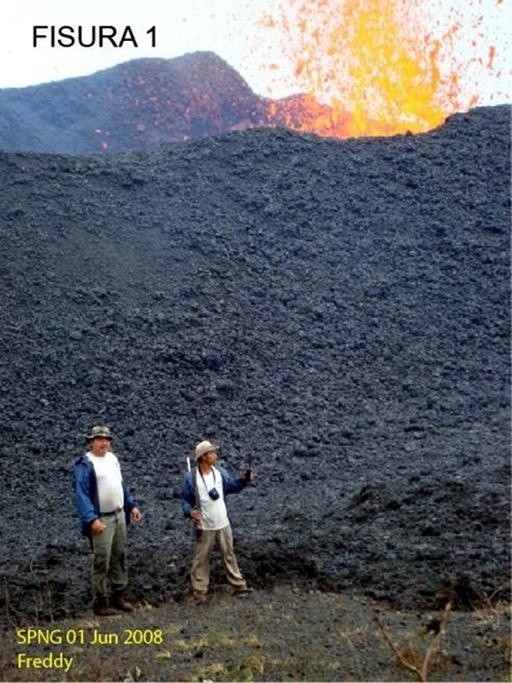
[99,508,123,517]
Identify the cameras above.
[208,488,219,500]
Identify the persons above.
[71,425,142,615]
[179,439,254,607]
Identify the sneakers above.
[231,586,254,598]
[193,592,207,605]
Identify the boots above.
[92,598,116,616]
[110,590,134,612]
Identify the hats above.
[193,439,220,463]
[84,424,114,445]
